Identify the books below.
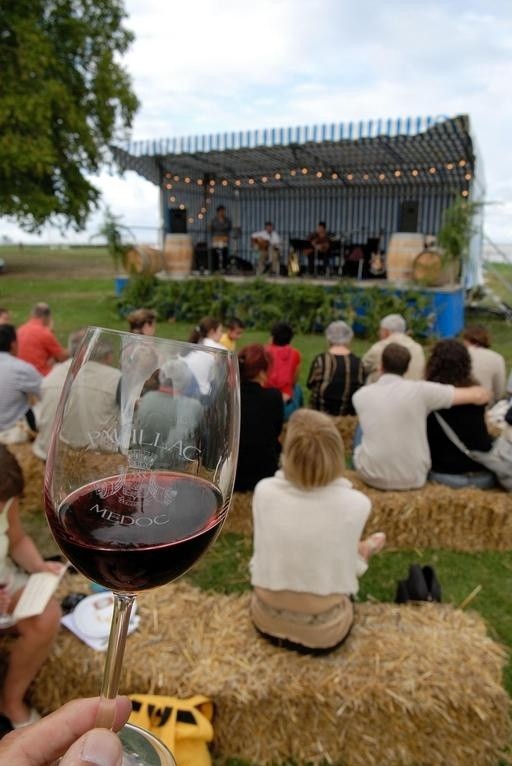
[1,562,70,630]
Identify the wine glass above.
[39,321,244,765]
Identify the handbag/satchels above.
[395,562,441,603]
[470,435,511,488]
[127,695,215,766]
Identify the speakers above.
[167,208,187,233]
[397,201,418,232]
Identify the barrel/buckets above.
[386,232,425,286]
[123,245,162,275]
[415,242,461,288]
[162,233,193,278]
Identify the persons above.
[250,410,385,657]
[251,222,282,276]
[0,693,135,763]
[308,220,330,276]
[211,204,233,273]
[1,444,66,729]
[1,303,512,491]
